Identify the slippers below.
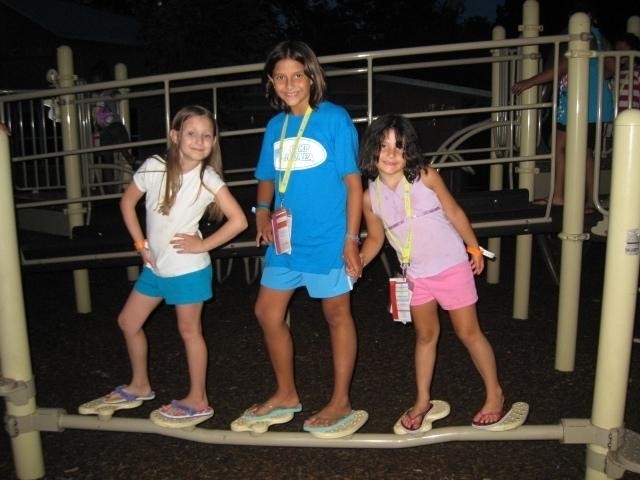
[401,403,434,431]
[531,196,564,208]
[244,398,304,421]
[583,204,598,217]
[472,400,513,430]
[158,399,215,419]
[302,407,358,433]
[103,383,158,405]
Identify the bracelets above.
[465,244,485,257]
[344,231,362,247]
[133,238,149,255]
[254,201,272,215]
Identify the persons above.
[87,99,142,196]
[339,112,509,431]
[509,9,616,218]
[100,105,251,419]
[598,31,640,210]
[243,39,363,431]
